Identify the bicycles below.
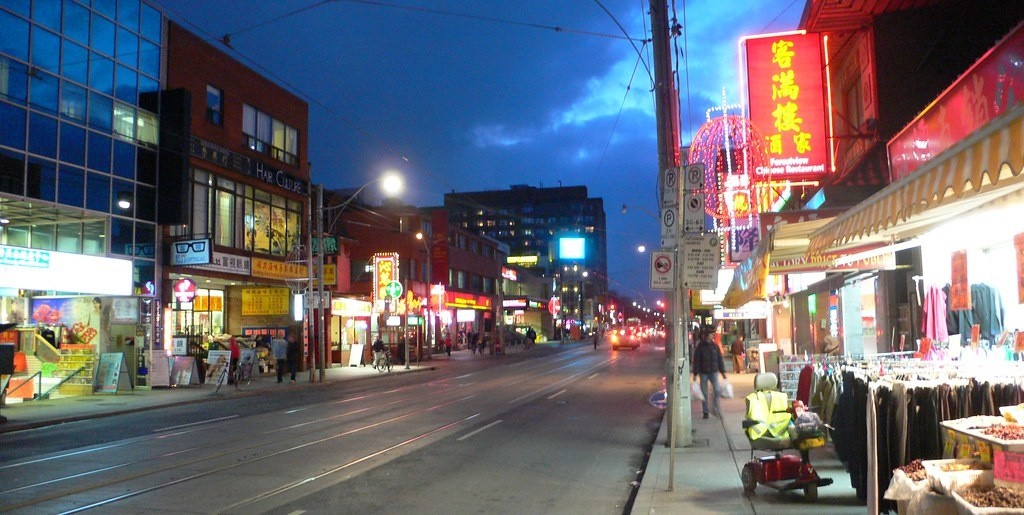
[376,351,395,372]
[214,356,253,392]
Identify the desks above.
[940,421,1024,463]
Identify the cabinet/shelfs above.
[209,336,273,373]
[58,354,94,395]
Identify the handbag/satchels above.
[690,381,705,401]
[719,378,733,399]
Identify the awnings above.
[720,215,896,311]
[804,101,1024,254]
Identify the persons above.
[442,323,449,336]
[445,332,452,357]
[271,329,288,384]
[525,326,536,344]
[691,324,727,419]
[87,297,107,359]
[372,339,391,372]
[229,337,239,371]
[730,334,747,373]
[246,227,257,250]
[285,335,300,384]
[467,330,487,354]
[397,338,405,364]
[591,325,605,349]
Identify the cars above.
[610,318,666,351]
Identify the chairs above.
[742,372,796,449]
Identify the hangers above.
[812,354,1024,390]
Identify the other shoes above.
[703,413,708,418]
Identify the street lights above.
[317,175,402,381]
[416,233,448,358]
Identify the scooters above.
[738,399,837,505]
